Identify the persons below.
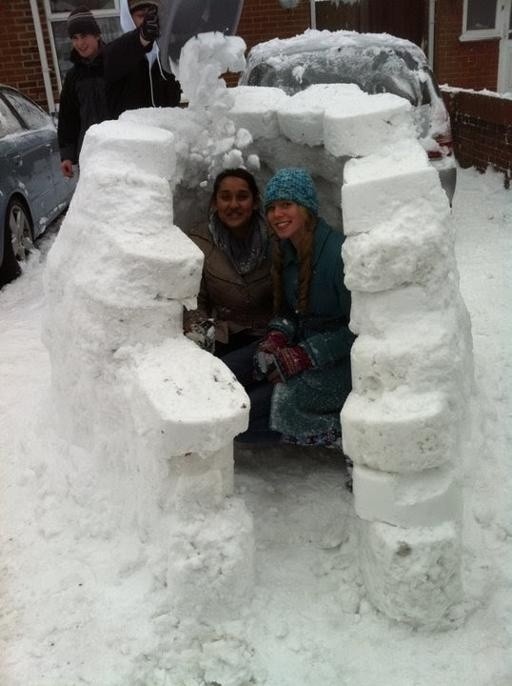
[184,167,283,356]
[56,7,117,179]
[219,166,357,448]
[103,0,181,110]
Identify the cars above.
[0,84,78,289]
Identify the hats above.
[65,4,102,36]
[259,165,322,215]
[127,0,160,11]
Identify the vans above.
[234,26,458,208]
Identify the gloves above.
[253,320,294,376]
[271,340,317,384]
[137,5,160,42]
